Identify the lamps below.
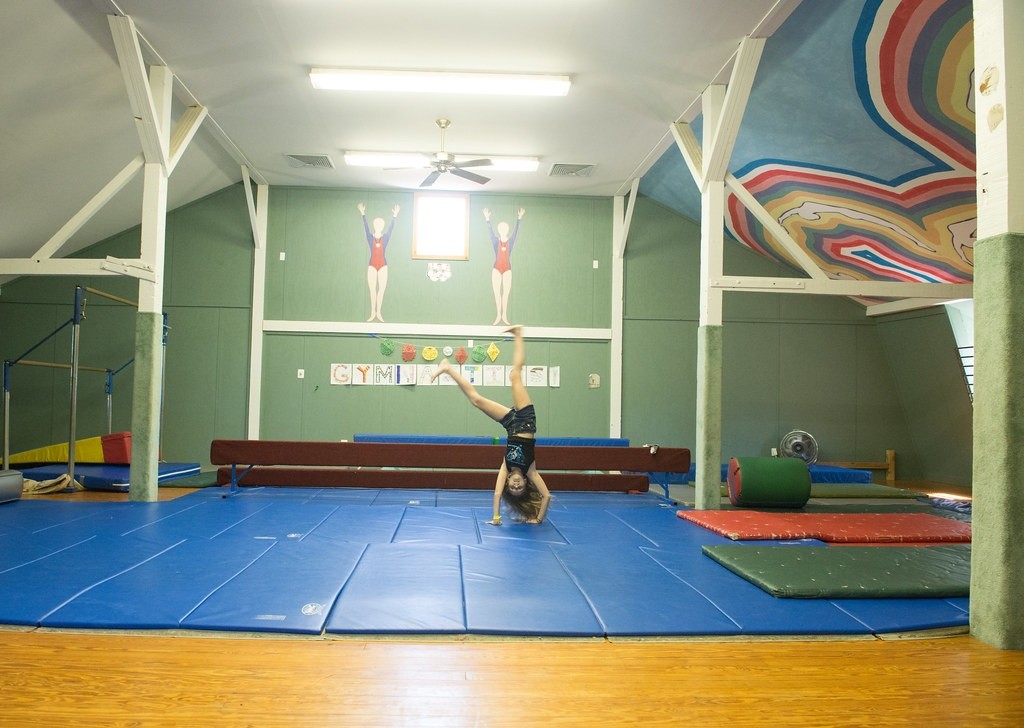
[310,68,570,98]
[344,151,539,172]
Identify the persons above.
[431,325,552,524]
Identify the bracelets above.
[536,518,542,523]
[493,515,501,520]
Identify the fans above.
[382,118,493,187]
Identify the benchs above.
[210,440,690,506]
